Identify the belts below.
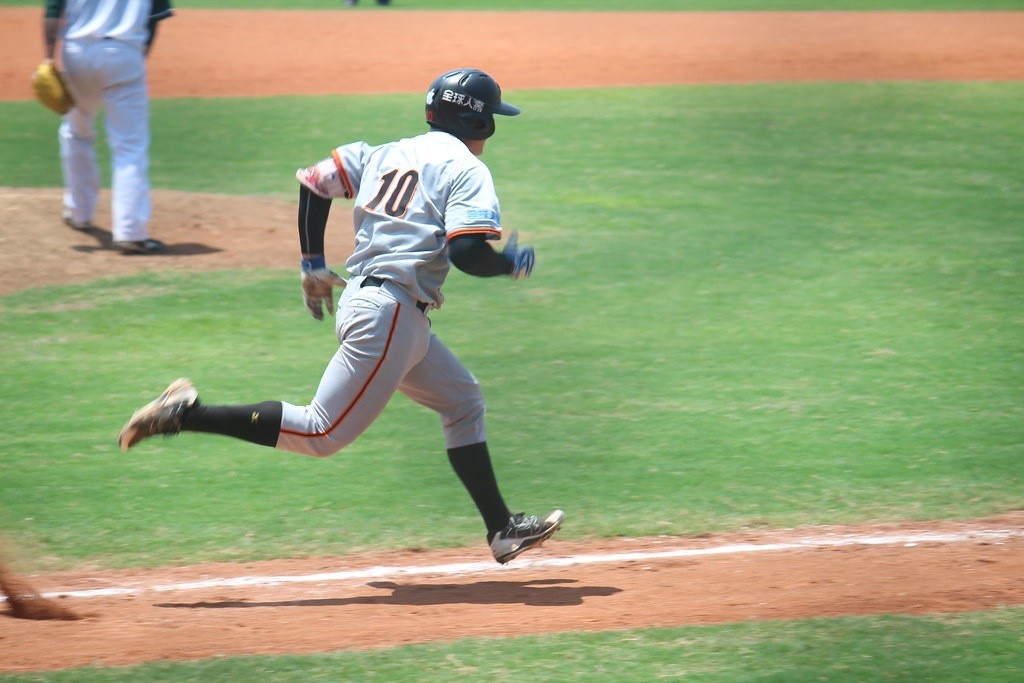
[360,276,429,313]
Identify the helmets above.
[425,69,521,140]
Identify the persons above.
[118,68,567,565]
[347,0,390,6]
[32,0,174,255]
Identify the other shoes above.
[117,239,166,253]
[62,210,90,229]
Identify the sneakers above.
[117,378,199,452]
[487,509,567,565]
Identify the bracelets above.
[301,256,326,271]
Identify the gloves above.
[501,230,535,280]
[300,255,348,321]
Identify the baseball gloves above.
[29,62,78,115]
